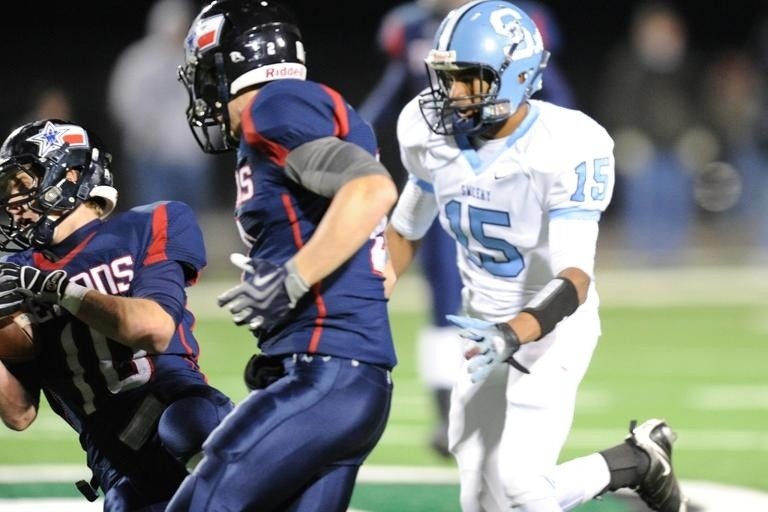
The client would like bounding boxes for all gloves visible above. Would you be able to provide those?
[216,253,310,338]
[445,314,531,384]
[0,262,70,319]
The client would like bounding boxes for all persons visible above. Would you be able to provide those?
[1,1,768,270]
[165,0,397,512]
[382,0,686,510]
[0,115,236,512]
[358,0,576,457]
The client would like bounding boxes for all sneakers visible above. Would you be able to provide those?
[625,419,687,512]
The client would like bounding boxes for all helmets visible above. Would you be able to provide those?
[184,2,308,98]
[424,1,550,124]
[0,119,118,219]
